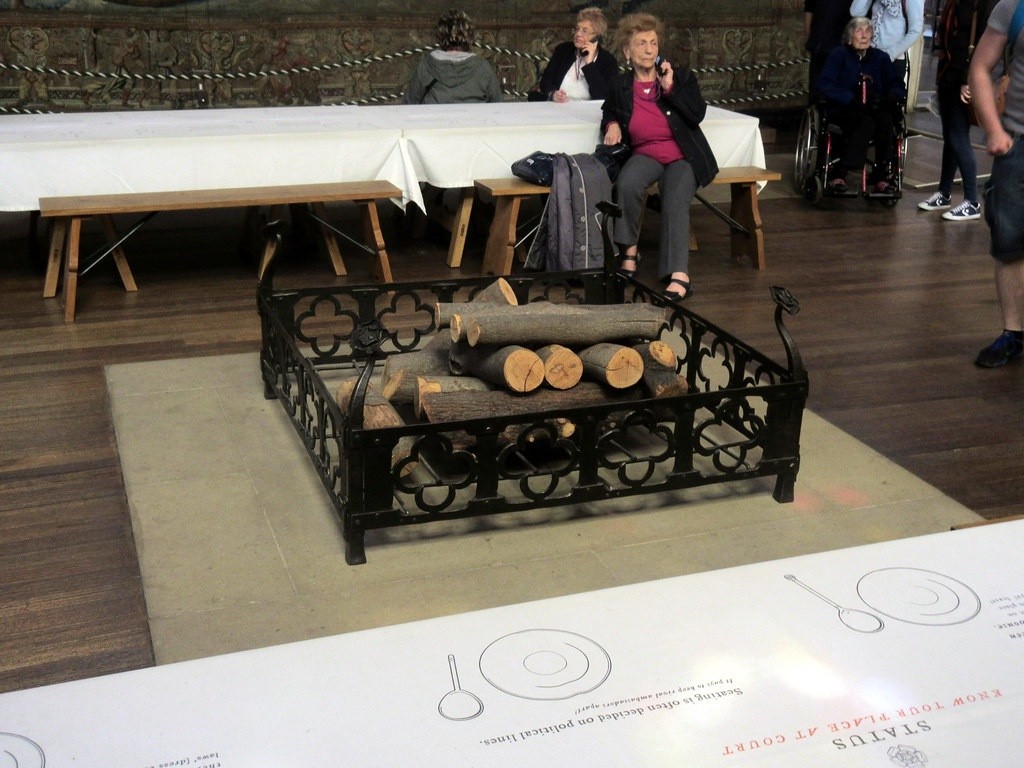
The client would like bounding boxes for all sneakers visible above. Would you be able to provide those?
[942,200,981,221]
[918,192,953,210]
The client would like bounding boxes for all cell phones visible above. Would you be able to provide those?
[655,55,668,77]
[579,34,603,57]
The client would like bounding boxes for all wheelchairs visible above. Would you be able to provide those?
[790,100,908,207]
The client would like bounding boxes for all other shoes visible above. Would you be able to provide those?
[874,181,895,194]
[972,330,1024,367]
[833,178,848,192]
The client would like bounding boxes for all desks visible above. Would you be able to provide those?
[403,100,770,268]
[0,104,428,301]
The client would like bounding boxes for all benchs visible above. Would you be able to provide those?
[37,180,403,323]
[473,164,782,279]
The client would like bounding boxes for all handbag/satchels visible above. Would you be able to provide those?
[511,151,552,186]
[940,57,970,95]
[970,75,1009,126]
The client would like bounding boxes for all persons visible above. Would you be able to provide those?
[408,9,502,104]
[918,0,991,220]
[973,0,1024,366]
[803,0,924,193]
[539,7,618,104]
[601,13,720,301]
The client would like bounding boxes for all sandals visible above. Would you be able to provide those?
[661,279,693,302]
[615,249,641,285]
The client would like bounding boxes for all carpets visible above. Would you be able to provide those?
[102,317,997,667]
